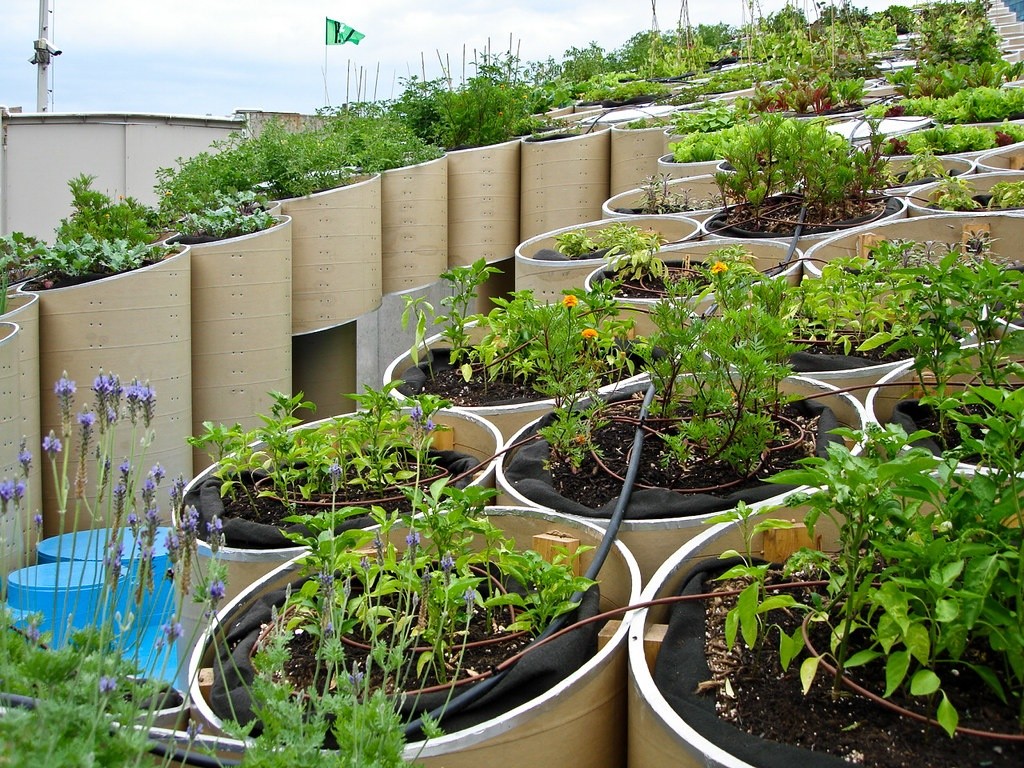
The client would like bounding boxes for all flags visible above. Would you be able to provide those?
[325,17,365,45]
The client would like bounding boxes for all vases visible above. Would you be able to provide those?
[491,366,871,591]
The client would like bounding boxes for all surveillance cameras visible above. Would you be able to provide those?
[42,39,63,56]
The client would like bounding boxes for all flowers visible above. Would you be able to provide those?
[0,366,478,768]
[511,242,847,482]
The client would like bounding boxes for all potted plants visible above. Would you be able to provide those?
[0,0,1024,768]
